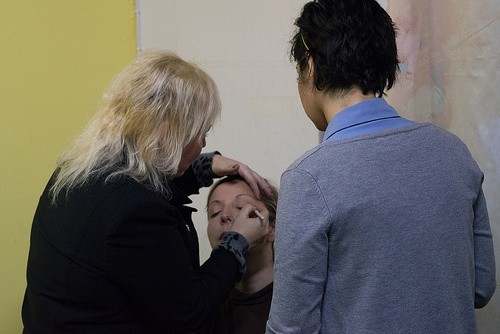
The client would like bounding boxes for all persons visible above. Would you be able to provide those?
[269,0,498,332]
[206,172,284,334]
[21,47,273,334]
